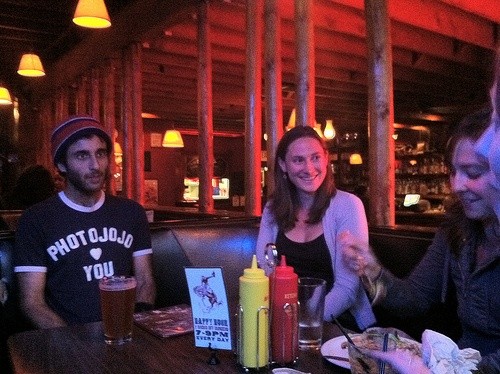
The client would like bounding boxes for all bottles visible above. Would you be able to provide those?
[395,179,452,195]
[403,158,448,173]
[268,255,299,361]
[239,254,269,368]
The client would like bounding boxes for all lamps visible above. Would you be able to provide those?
[72,0,112,29]
[0,87,13,105]
[162,124,184,148]
[17,35,46,78]
[284,107,363,166]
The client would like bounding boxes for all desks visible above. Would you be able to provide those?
[4,304,358,374]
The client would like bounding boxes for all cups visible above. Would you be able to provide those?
[99,278,137,345]
[348,334,398,374]
[298,278,327,351]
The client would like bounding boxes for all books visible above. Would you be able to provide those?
[132,302,193,341]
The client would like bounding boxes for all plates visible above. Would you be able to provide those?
[321,334,422,370]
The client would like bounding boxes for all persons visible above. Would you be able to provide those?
[366,44,500,374]
[338,101,500,346]
[256,125,377,333]
[12,113,156,330]
[0,135,58,209]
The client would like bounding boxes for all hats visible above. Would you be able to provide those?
[49,114,113,161]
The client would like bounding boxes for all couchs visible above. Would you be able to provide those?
[0,221,462,374]
